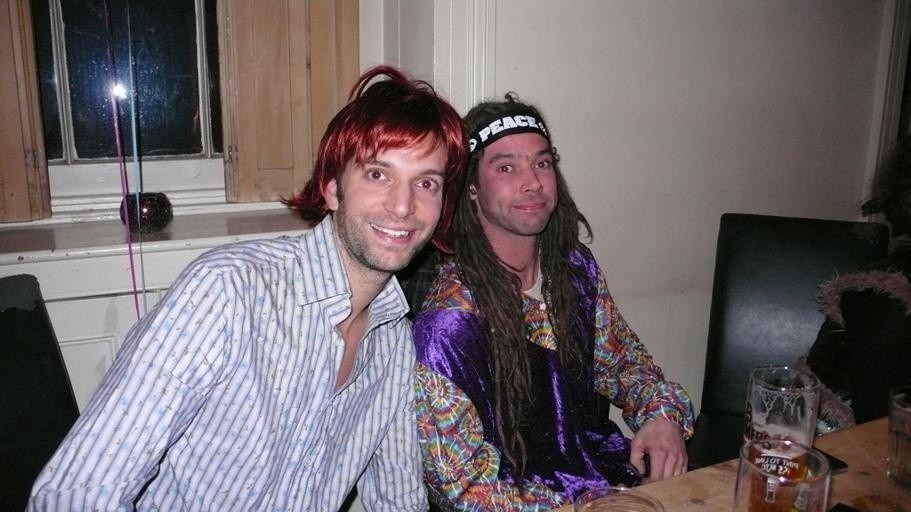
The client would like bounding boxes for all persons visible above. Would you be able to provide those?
[24,57,470,512]
[406,90,696,512]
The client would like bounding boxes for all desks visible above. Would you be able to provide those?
[538,407,911,512]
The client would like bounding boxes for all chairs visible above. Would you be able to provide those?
[1,274,83,511]
[685,212,891,471]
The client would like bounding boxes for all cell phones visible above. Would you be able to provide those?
[805,445,848,477]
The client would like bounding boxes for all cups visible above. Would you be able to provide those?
[884,383,910,490]
[732,366,832,512]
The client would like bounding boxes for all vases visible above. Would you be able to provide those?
[120,192,174,234]
